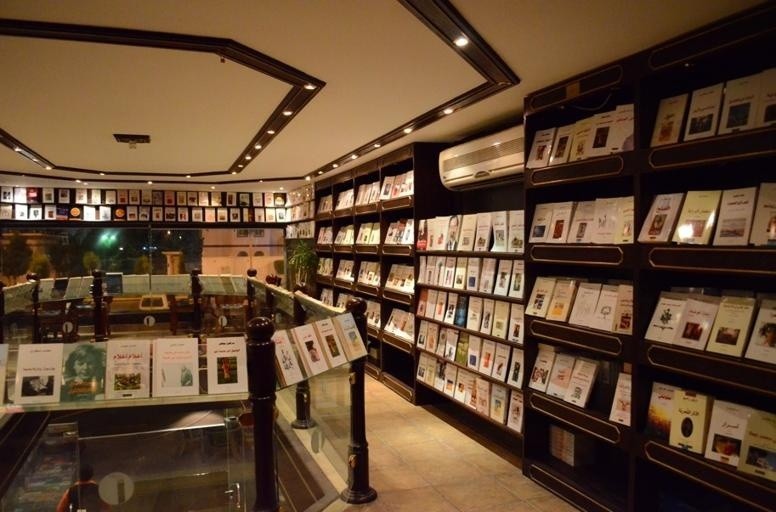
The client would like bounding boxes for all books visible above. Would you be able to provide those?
[525,275,633,336]
[643,286,776,364]
[416,211,526,299]
[528,198,634,246]
[317,257,415,345]
[636,183,774,248]
[645,379,774,481]
[526,104,634,169]
[647,72,776,149]
[152,338,248,396]
[105,272,248,296]
[414,288,525,433]
[248,278,346,319]
[270,313,369,388]
[2,277,94,314]
[15,340,151,403]
[316,170,414,244]
[548,424,575,467]
[526,343,632,428]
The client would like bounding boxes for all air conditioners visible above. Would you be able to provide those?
[439,125,523,191]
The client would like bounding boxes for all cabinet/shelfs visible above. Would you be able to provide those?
[520,53,641,511]
[412,244,524,472]
[639,3,776,511]
[318,149,475,406]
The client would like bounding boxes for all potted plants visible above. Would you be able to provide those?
[289,240,318,322]
